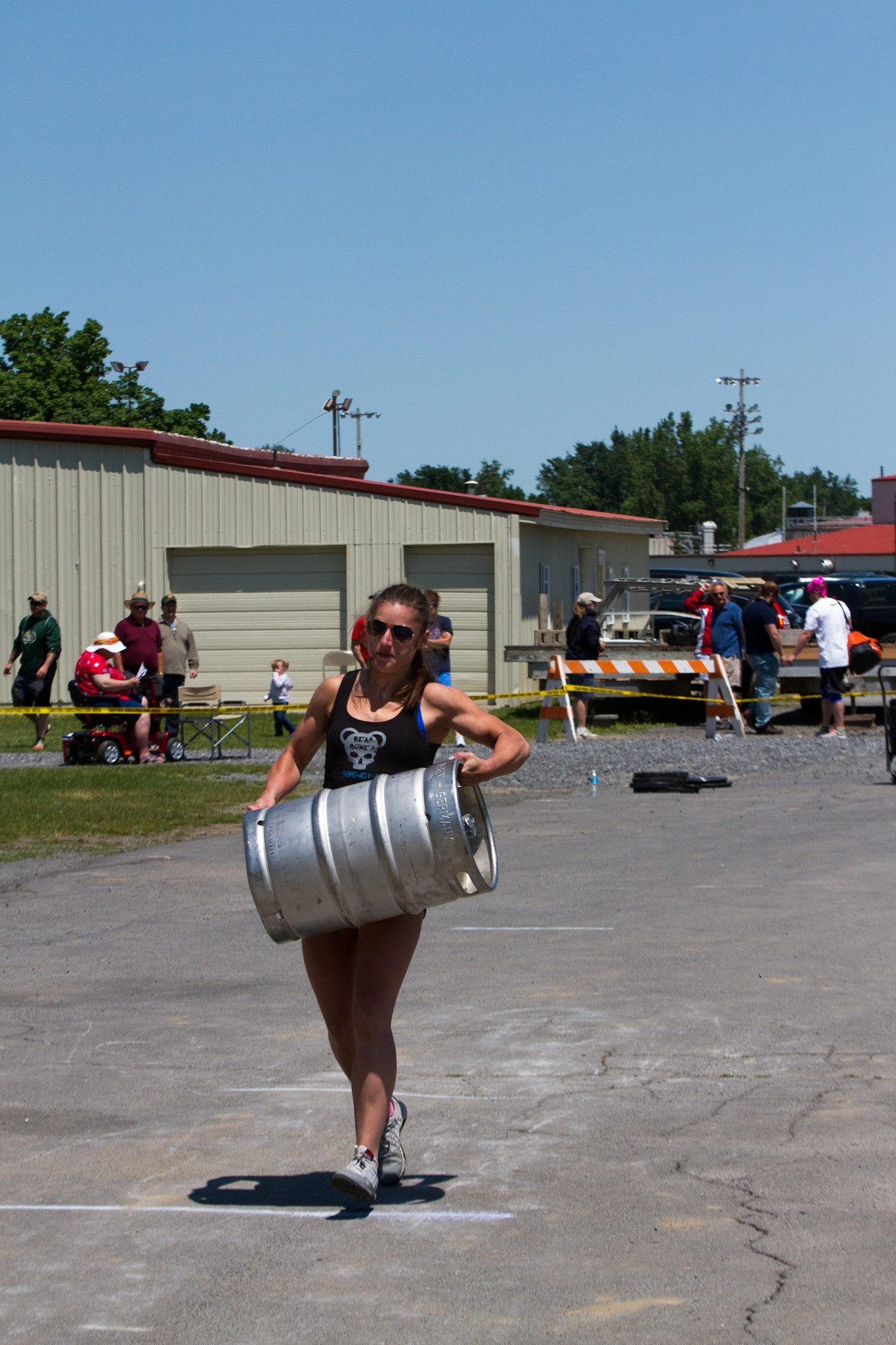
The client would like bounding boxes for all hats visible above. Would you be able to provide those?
[161,595,177,604]
[27,591,46,602]
[369,590,383,599]
[86,632,127,653]
[576,592,602,605]
[124,592,155,610]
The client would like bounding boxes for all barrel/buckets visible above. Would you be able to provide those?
[241,758,498,945]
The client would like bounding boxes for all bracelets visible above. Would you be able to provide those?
[158,672,164,675]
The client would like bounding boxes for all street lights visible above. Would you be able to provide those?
[323,389,354,456]
[715,368,764,551]
[340,408,382,459]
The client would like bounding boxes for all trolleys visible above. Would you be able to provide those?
[877,663,896,784]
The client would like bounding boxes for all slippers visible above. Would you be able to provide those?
[139,753,166,764]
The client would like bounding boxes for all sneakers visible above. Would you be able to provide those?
[332,1145,378,1212]
[819,726,847,739]
[379,1096,407,1182]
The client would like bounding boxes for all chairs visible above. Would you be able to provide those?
[179,686,254,764]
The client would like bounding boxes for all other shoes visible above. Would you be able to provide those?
[289,729,295,737]
[757,721,783,735]
[455,735,465,747]
[46,723,51,731]
[577,728,598,739]
[274,734,283,737]
[31,739,44,750]
[716,716,748,730]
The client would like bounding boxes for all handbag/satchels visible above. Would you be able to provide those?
[848,631,883,676]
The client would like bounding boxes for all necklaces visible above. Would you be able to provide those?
[371,680,394,699]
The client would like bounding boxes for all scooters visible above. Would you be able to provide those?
[61,679,184,765]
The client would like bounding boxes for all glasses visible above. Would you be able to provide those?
[429,603,438,608]
[132,605,148,610]
[30,600,45,606]
[365,619,425,644]
[712,592,724,597]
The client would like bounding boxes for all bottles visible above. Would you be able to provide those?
[589,770,598,797]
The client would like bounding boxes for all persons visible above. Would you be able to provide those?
[565,591,605,737]
[74,593,199,765]
[685,577,746,729]
[786,576,852,738]
[3,591,61,750]
[741,581,785,735]
[352,589,466,749]
[263,660,297,737]
[247,584,531,1202]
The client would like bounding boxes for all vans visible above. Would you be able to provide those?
[618,566,896,641]
